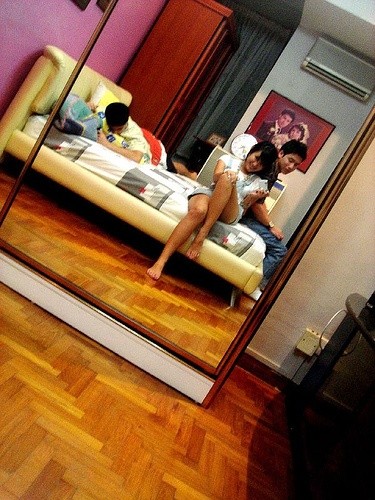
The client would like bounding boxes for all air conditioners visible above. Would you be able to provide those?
[301,36,375,102]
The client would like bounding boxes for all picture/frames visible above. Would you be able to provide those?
[206,133,226,148]
[244,90,336,174]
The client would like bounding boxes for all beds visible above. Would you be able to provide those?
[0,45,263,307]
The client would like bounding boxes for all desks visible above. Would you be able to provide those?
[284,293,375,411]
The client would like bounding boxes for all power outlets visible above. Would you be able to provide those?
[295,328,328,357]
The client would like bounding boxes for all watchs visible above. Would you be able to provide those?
[266,220,275,229]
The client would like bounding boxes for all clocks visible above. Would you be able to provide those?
[230,133,258,160]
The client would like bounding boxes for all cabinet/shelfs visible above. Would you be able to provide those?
[118,0,238,158]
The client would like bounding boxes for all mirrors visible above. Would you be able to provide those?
[1,1,375,407]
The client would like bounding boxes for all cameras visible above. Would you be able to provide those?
[259,180,269,194]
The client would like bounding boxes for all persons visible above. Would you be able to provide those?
[87,101,198,182]
[272,121,309,153]
[240,139,308,299]
[146,140,278,281]
[254,108,296,142]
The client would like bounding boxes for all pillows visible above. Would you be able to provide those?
[89,81,120,112]
